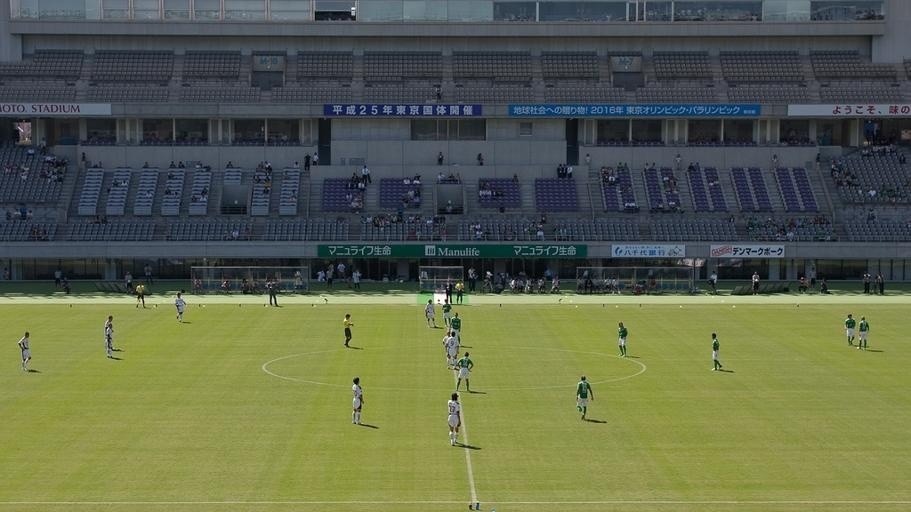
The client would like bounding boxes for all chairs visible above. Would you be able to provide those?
[1,41,911,295]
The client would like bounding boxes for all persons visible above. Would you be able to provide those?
[106,322,114,359]
[617,319,629,358]
[424,298,476,392]
[846,313,857,346]
[576,374,595,421]
[857,315,871,350]
[0,108,911,308]
[18,331,32,373]
[710,332,724,372]
[103,315,115,349]
[350,375,365,424]
[343,312,356,348]
[175,292,187,324]
[447,392,463,446]
